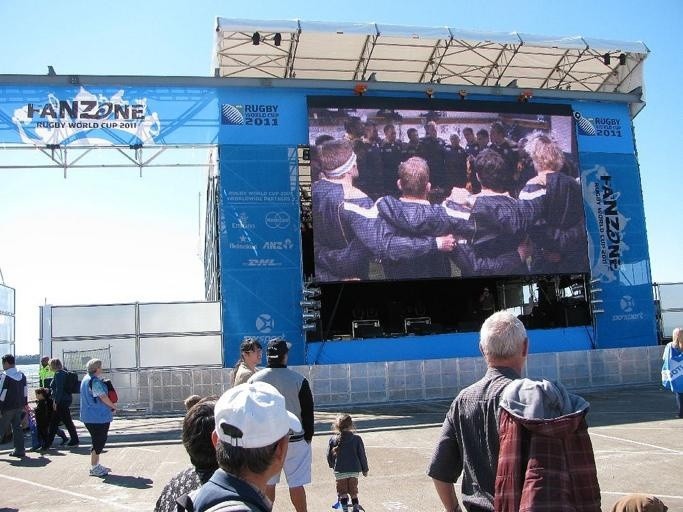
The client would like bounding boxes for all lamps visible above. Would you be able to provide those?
[604,53,611,65]
[519,90,534,103]
[589,276,606,316]
[355,82,368,96]
[620,54,627,65]
[299,281,322,333]
[252,32,260,45]
[273,33,281,46]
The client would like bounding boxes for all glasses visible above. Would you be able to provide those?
[246,349,262,353]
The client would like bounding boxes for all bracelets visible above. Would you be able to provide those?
[453,503,459,512]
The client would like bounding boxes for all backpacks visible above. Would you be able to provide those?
[89,379,118,403]
[54,370,80,393]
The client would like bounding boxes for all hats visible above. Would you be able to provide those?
[214,381,302,449]
[86,359,101,372]
[267,338,292,358]
[612,495,668,512]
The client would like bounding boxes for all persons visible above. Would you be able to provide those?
[79,358,120,476]
[154,394,221,512]
[0,352,80,458]
[660,327,683,419]
[185,395,202,411]
[232,338,262,388]
[312,115,590,285]
[326,413,369,512]
[172,381,302,512]
[247,336,314,511]
[424,310,603,512]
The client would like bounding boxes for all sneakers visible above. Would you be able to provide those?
[89,464,113,476]
[35,447,48,452]
[9,451,25,456]
[59,439,69,446]
[67,440,79,447]
[30,447,38,451]
